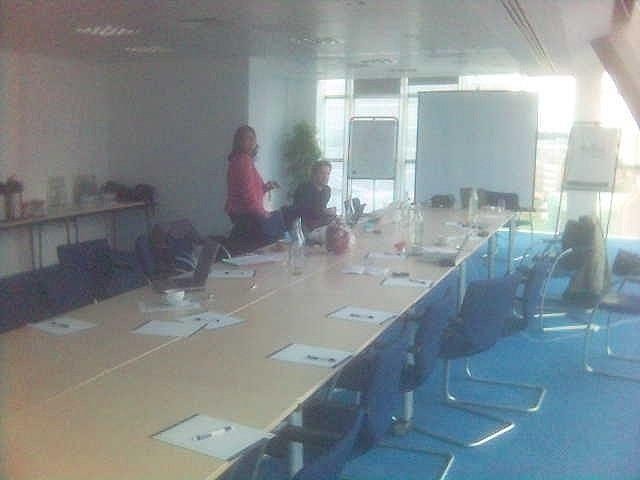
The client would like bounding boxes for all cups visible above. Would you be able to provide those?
[164,288,185,305]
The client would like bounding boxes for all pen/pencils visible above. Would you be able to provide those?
[225,272,246,275]
[350,314,375,318]
[197,426,232,440]
[307,355,336,361]
[409,279,426,284]
[48,321,70,328]
[195,318,221,322]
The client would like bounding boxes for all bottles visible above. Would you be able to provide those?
[287,215,306,277]
[468,187,480,221]
[400,198,424,256]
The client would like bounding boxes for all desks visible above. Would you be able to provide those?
[2,192,515,479]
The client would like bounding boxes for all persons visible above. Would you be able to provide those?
[223,124,281,251]
[293,159,336,231]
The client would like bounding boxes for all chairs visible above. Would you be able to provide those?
[583,251,640,381]
[442,276,547,412]
[238,404,365,479]
[399,291,520,446]
[522,245,599,337]
[319,337,451,479]
[1,192,337,331]
